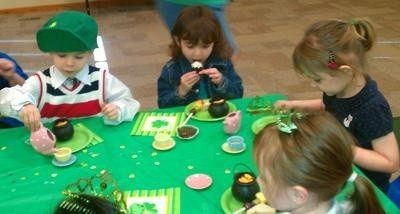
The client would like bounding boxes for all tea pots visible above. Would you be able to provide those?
[29,122,59,155]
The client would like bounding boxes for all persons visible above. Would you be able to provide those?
[154,0,236,55]
[53,191,128,214]
[157,4,244,110]
[274,17,400,196]
[251,109,387,214]
[0,9,141,133]
[0,51,29,128]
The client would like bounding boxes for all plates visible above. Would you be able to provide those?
[251,115,286,134]
[185,173,213,190]
[55,126,93,153]
[221,187,248,214]
[222,143,246,154]
[52,155,77,167]
[152,138,176,150]
[184,98,235,122]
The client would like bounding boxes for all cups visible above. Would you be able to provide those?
[227,136,244,150]
[154,134,171,148]
[56,148,71,161]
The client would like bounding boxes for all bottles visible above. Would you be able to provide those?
[233,172,259,204]
[52,118,73,142]
[208,96,229,118]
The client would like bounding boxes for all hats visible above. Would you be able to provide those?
[36,10,99,53]
